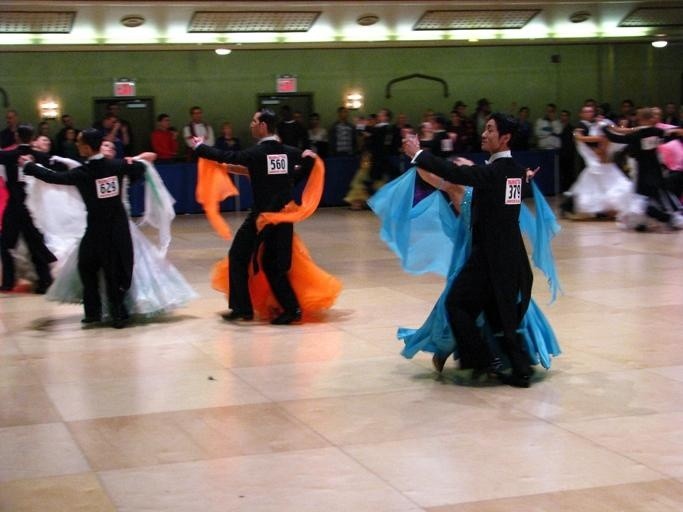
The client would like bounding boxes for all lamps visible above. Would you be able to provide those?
[215,49,231,55]
[40,103,58,119]
[651,40,668,48]
[346,94,363,110]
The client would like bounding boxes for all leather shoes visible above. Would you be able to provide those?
[221,309,253,320]
[433,350,534,388]
[271,308,301,324]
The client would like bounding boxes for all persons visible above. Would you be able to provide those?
[152,99,537,213]
[0,133,88,281]
[366,133,562,370]
[193,107,316,324]
[0,112,130,235]
[535,98,683,236]
[17,127,146,330]
[192,136,343,322]
[0,122,69,294]
[403,110,533,388]
[17,134,198,323]
